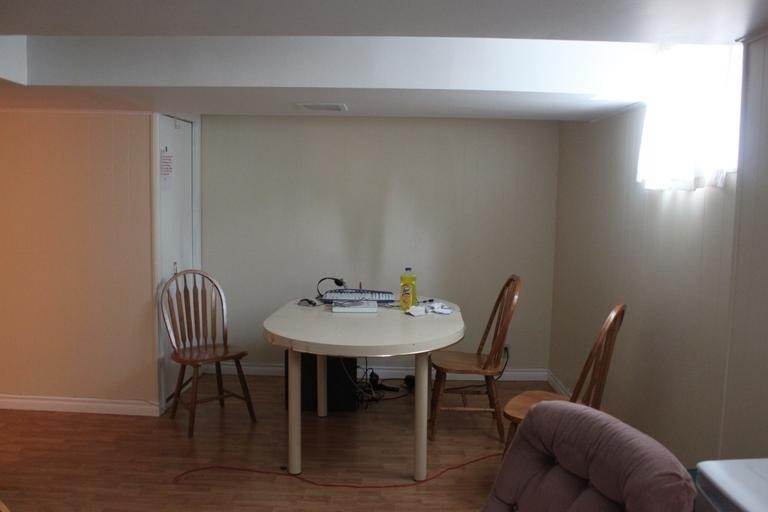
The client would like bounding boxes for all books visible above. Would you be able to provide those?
[332,299,378,313]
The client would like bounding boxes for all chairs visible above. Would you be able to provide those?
[428,272,630,461]
[476,396,699,512]
[158,268,257,441]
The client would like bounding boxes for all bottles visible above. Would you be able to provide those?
[399,266,419,312]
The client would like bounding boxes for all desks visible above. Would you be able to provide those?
[262,292,467,485]
